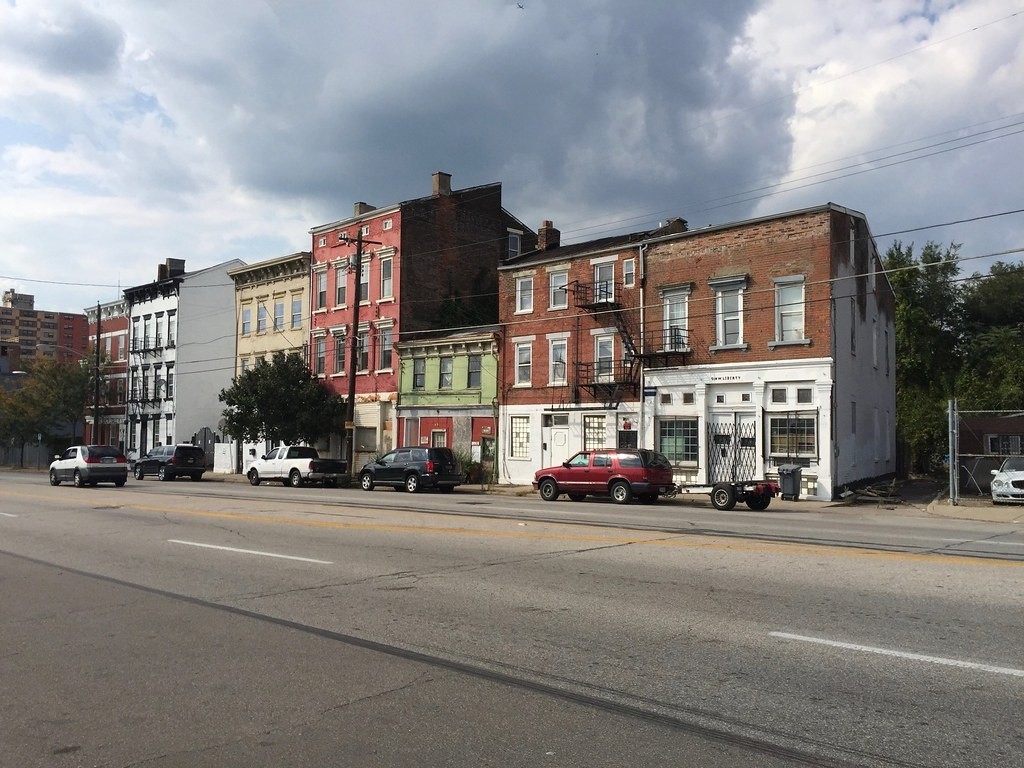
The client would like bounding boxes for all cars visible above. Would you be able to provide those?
[990,457,1024,505]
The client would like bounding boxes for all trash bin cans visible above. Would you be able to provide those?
[777,463,804,501]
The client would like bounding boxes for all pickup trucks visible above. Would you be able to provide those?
[246,444,349,489]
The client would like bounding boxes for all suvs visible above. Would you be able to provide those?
[357,445,463,493]
[133,444,208,481]
[531,448,677,505]
[49,445,128,488]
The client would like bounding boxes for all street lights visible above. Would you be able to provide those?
[36,343,101,445]
